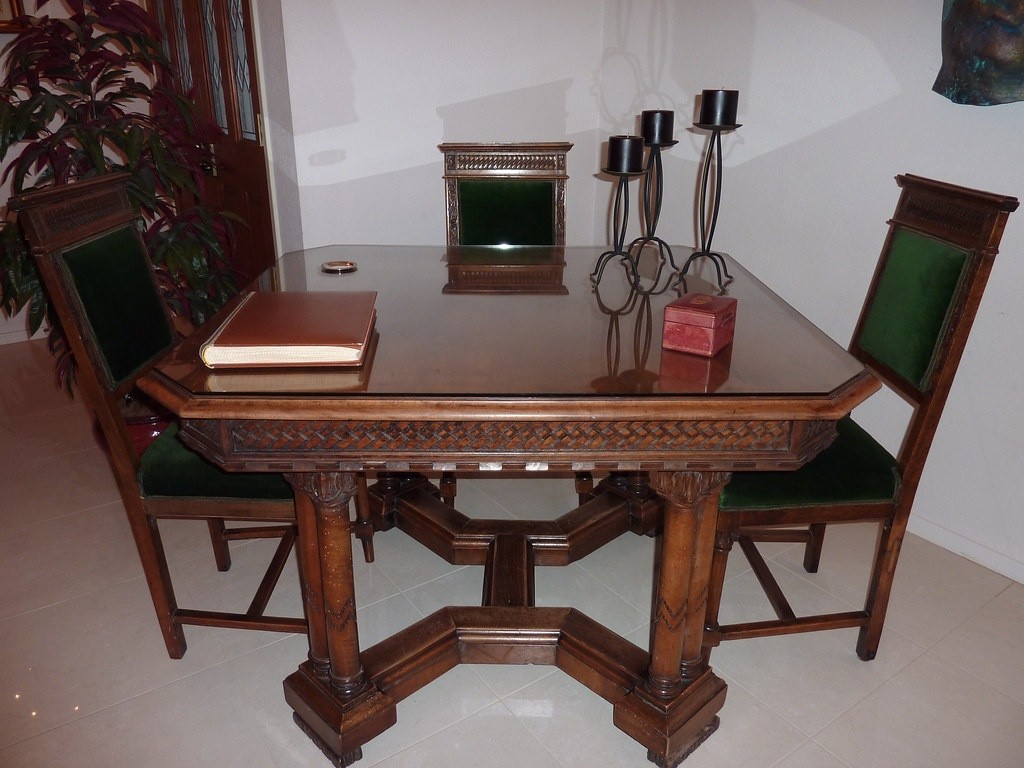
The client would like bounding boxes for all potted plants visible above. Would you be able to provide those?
[1,0,259,462]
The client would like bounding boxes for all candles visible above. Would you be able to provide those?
[608,132,643,172]
[639,109,675,144]
[700,87,740,125]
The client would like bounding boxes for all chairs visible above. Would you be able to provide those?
[439,137,595,510]
[12,171,373,661]
[701,170,1019,671]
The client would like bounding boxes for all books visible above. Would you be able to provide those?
[199,290,378,369]
[204,329,381,394]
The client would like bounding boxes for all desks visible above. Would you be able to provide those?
[137,241,884,768]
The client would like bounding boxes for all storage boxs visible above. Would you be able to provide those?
[663,289,735,358]
